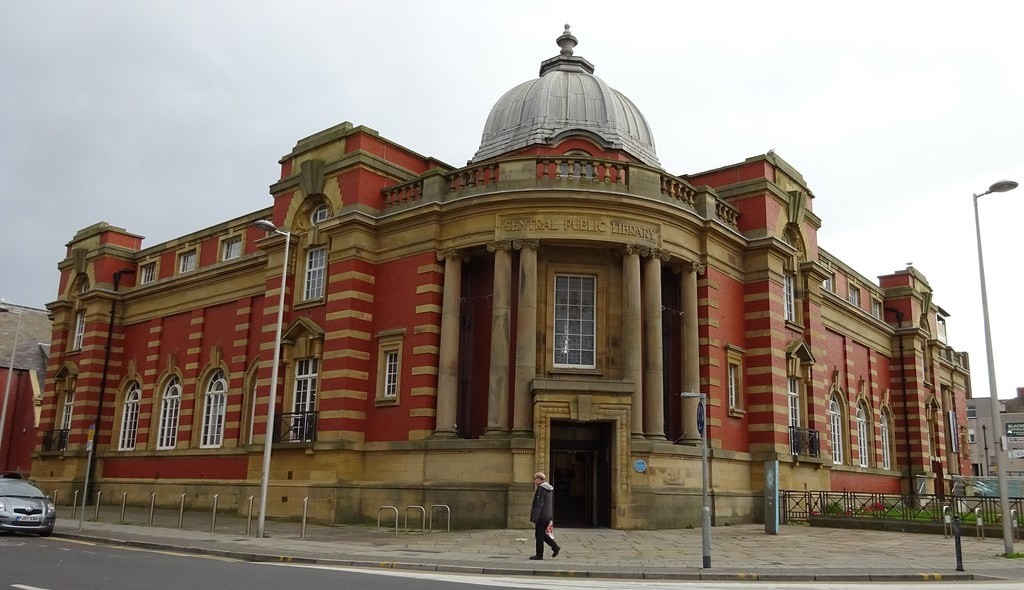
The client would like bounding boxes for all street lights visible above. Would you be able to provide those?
[973,180,1018,555]
[0,307,22,450]
[250,220,291,538]
[682,393,712,568]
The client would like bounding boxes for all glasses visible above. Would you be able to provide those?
[534,477,539,480]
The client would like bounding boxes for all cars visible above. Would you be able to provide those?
[0,478,56,537]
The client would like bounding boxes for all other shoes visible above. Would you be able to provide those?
[552,546,561,558]
[529,555,543,560]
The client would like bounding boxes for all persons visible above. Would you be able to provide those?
[529,472,561,561]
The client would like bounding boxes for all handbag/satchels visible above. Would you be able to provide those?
[545,520,555,539]
